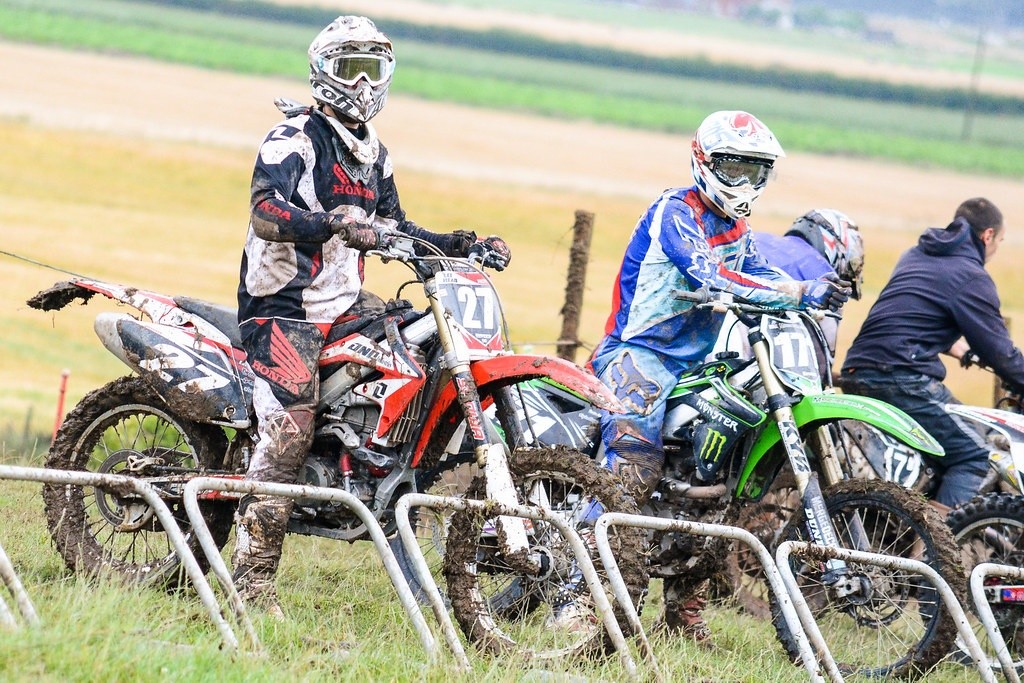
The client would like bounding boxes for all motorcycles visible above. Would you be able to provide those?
[25,215,653,674]
[440,284,1024,683]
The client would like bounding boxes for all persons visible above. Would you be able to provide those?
[229,16,512,618]
[544,109,863,656]
[841,196,1024,565]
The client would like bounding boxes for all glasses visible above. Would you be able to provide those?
[308,51,396,87]
[692,131,773,189]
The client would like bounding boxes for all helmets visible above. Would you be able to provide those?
[691,110,785,222]
[783,208,864,301]
[307,15,395,124]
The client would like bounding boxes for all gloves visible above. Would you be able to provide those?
[796,270,852,313]
[331,214,380,251]
[462,230,511,272]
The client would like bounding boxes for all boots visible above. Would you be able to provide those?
[543,518,607,639]
[908,500,956,580]
[658,598,718,655]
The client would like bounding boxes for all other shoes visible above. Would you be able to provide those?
[226,575,284,623]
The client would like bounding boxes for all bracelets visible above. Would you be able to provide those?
[960,349,975,369]
